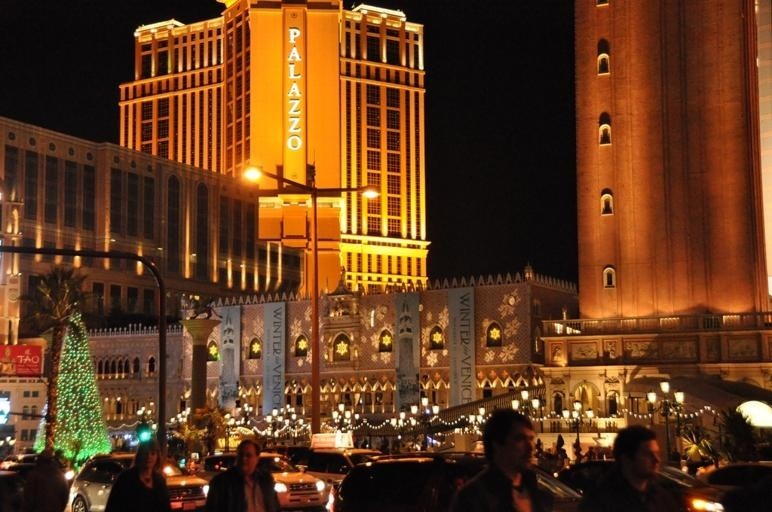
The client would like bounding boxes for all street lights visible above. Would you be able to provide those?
[246,166,381,434]
[563,398,594,451]
[136,405,153,425]
[512,388,540,418]
[386,398,440,453]
[332,399,361,433]
[0,435,17,455]
[169,406,191,431]
[220,411,234,449]
[648,380,684,462]
[470,406,485,443]
[263,403,304,448]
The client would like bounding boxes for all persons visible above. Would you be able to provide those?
[105,440,170,512]
[577,426,685,512]
[360,436,402,451]
[205,439,280,512]
[450,408,554,512]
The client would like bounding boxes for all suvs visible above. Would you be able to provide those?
[326,452,583,512]
[298,447,383,490]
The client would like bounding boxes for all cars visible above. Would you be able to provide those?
[187,452,329,509]
[554,458,744,512]
[0,453,40,472]
[694,459,772,488]
[71,453,209,512]
